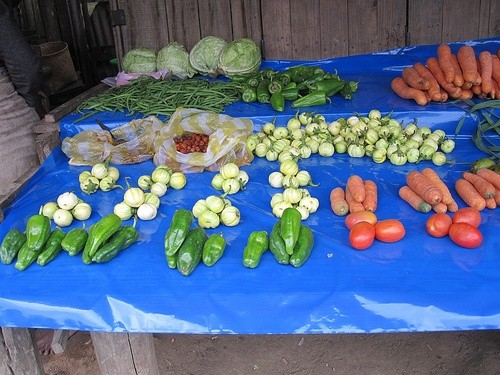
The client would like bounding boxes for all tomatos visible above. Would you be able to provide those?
[426,206,482,248]
[345,211,405,250]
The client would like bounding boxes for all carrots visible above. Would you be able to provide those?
[397,168,458,214]
[455,167,500,211]
[330,174,377,215]
[390,43,500,105]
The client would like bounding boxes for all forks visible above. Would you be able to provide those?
[96,119,125,145]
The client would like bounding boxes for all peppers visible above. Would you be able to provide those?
[242,204,315,268]
[227,65,358,112]
[0,204,139,271]
[162,208,226,276]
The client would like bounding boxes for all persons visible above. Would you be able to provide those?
[0,0,47,202]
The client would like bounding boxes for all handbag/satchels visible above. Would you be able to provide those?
[60,106,252,173]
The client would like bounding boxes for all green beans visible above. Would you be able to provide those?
[72,70,243,123]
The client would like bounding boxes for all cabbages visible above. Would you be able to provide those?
[122,36,261,79]
